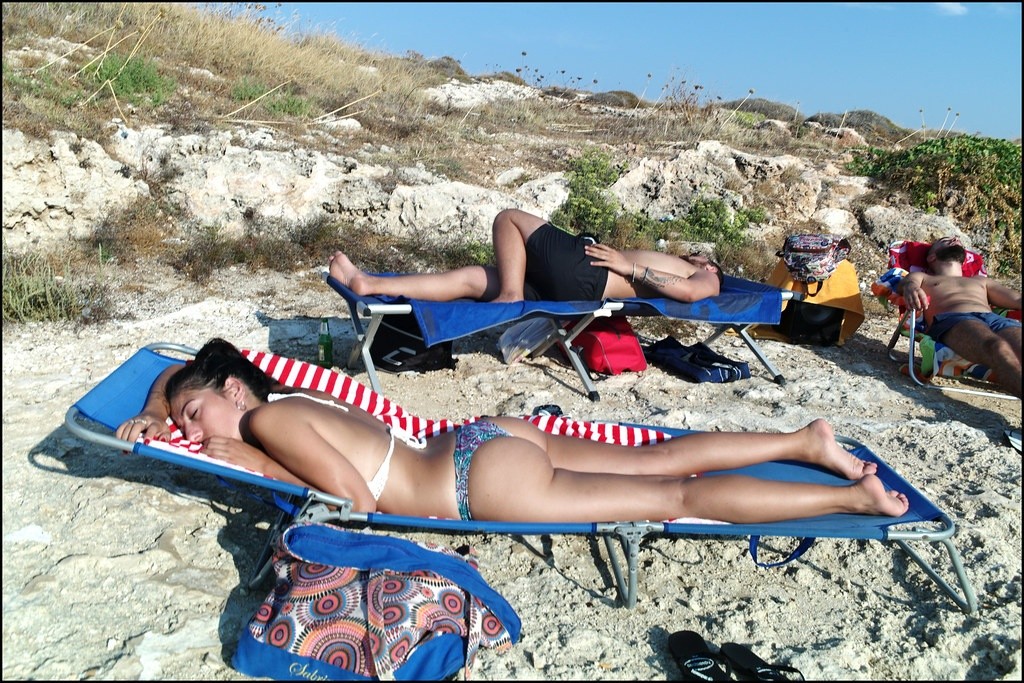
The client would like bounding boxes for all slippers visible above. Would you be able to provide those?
[668,630,806,683]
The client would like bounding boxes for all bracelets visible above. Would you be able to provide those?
[642,266,649,284]
[632,263,637,283]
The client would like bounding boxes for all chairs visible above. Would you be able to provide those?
[65,240,1022,616]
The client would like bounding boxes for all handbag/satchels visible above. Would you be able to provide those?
[561,318,646,375]
[774,235,851,298]
[230,524,522,681]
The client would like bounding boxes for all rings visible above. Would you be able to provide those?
[129,420,134,425]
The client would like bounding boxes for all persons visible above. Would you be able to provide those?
[903,236,1022,399]
[114,337,909,524]
[330,208,725,305]
